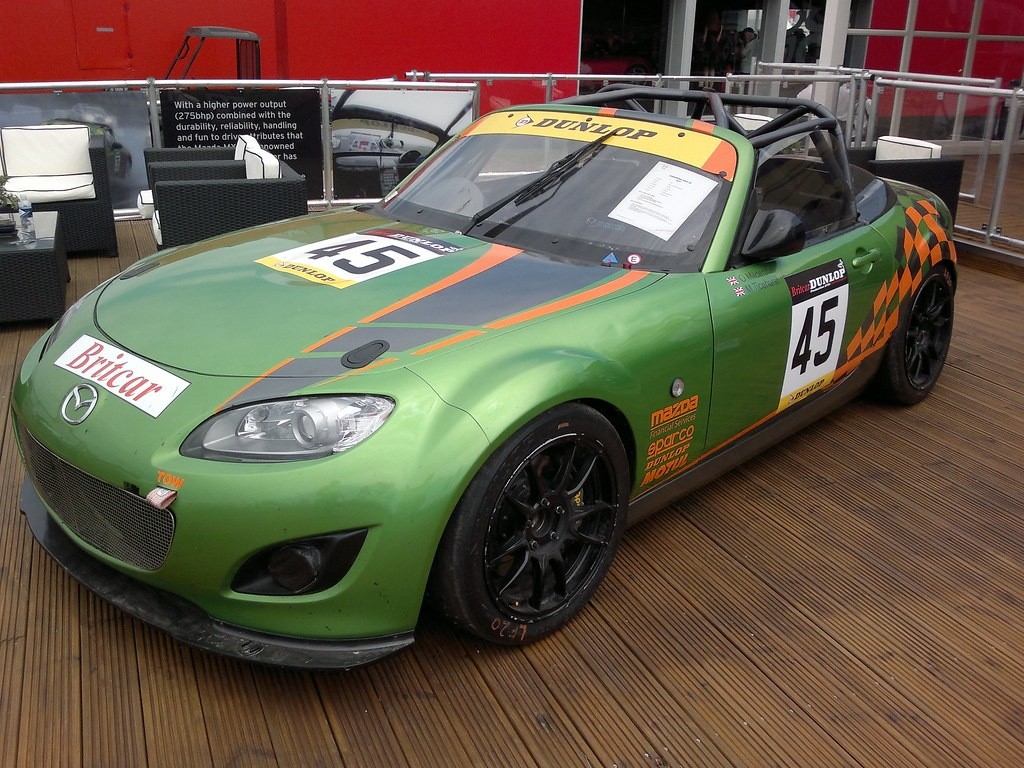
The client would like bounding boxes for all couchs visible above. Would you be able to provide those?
[136,134,308,251]
[0,124,120,259]
[808,135,966,232]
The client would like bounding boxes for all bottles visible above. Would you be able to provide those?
[18,194,36,241]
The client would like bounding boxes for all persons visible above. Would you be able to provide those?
[582,27,806,94]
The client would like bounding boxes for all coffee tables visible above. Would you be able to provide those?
[0,211,71,329]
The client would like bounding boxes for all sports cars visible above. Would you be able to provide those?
[7,83,961,670]
